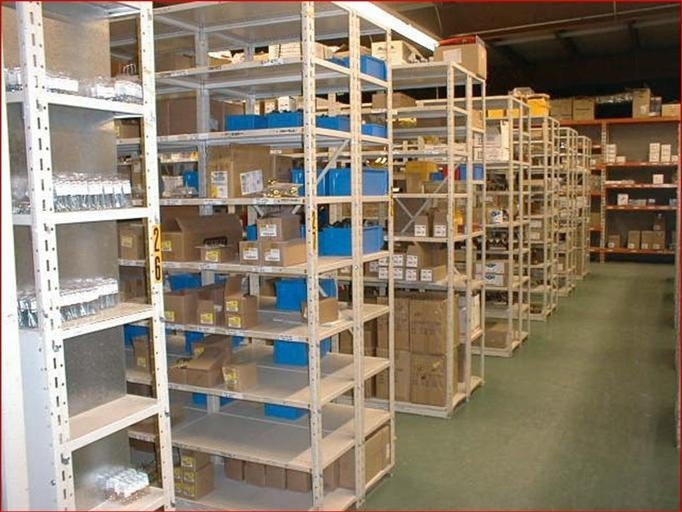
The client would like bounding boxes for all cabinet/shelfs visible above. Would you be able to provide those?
[1,0,395,512]
[376,58,592,419]
[551,88,682,265]
